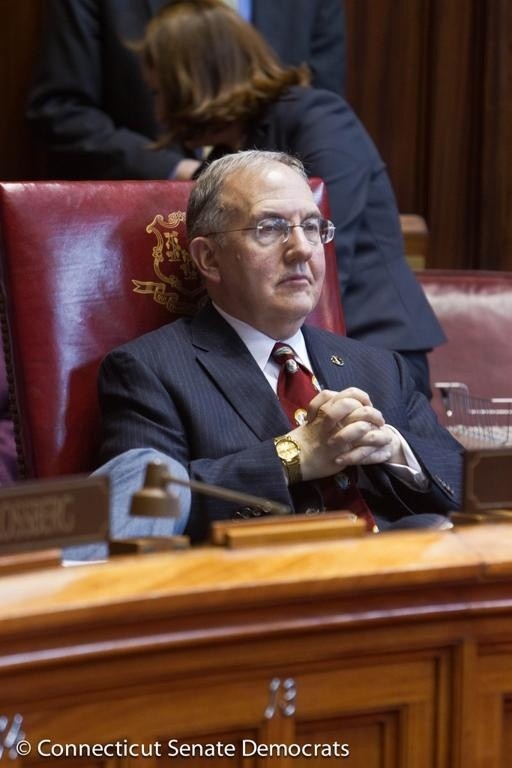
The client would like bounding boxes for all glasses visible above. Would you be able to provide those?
[202,215,337,249]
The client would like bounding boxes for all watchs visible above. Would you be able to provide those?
[273,433,305,487]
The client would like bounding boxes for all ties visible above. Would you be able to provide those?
[272,343,377,538]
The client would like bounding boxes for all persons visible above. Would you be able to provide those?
[8,1,347,179]
[91,148,474,556]
[122,0,448,406]
